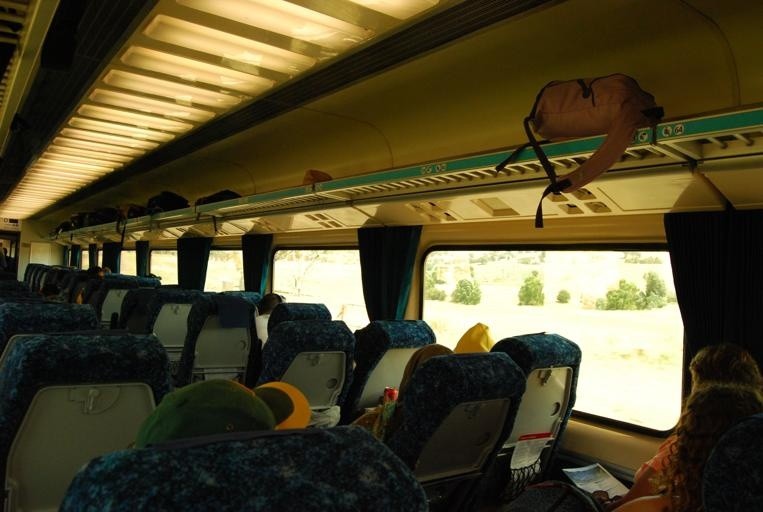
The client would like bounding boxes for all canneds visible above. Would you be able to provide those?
[384,386,399,404]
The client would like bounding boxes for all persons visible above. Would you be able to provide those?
[350,342,455,441]
[591,340,762,512]
[0,242,9,272]
[256,292,282,352]
[71,266,111,305]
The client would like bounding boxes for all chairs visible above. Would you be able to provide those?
[0,264,763,510]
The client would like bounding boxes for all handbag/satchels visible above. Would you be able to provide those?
[196,189,242,206]
[52,190,190,235]
[303,169,333,185]
[531,73,664,141]
